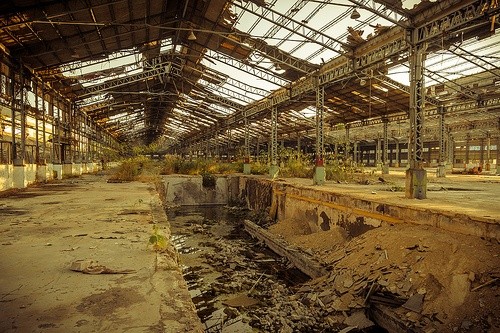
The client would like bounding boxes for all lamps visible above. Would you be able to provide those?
[350,7,361,19]
[187,30,197,40]
[360,79,366,86]
